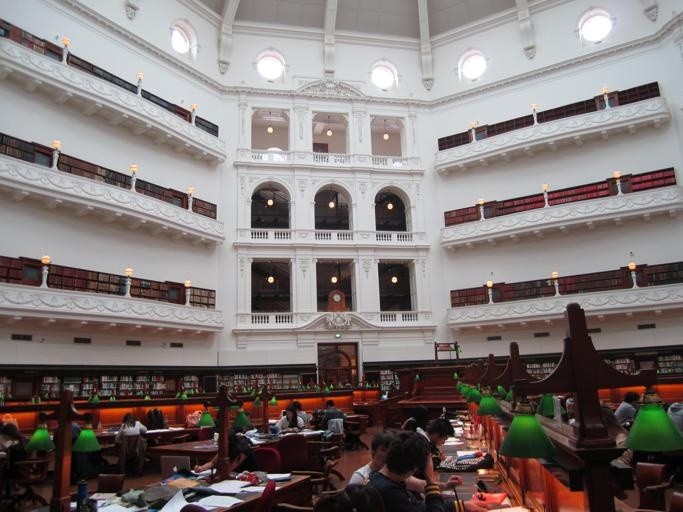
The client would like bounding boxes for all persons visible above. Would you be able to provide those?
[72,423,82,440]
[320,400,343,428]
[613,391,640,432]
[194,427,256,484]
[0,422,32,503]
[519,395,535,412]
[347,404,491,511]
[273,405,305,434]
[292,401,310,424]
[115,413,148,478]
[600,405,633,466]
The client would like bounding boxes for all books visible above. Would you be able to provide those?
[265,472,292,482]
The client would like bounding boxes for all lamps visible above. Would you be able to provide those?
[454,372,683,458]
[267,112,398,283]
[315,383,384,395]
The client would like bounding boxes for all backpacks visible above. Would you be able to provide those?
[146,407,165,430]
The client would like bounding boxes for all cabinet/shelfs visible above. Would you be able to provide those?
[438,81,661,152]
[444,166,676,228]
[0,132,217,219]
[0,255,216,309]
[0,17,218,138]
[450,261,683,308]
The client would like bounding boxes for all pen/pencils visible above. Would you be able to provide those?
[241,484,253,488]
[452,486,458,501]
[196,457,199,468]
